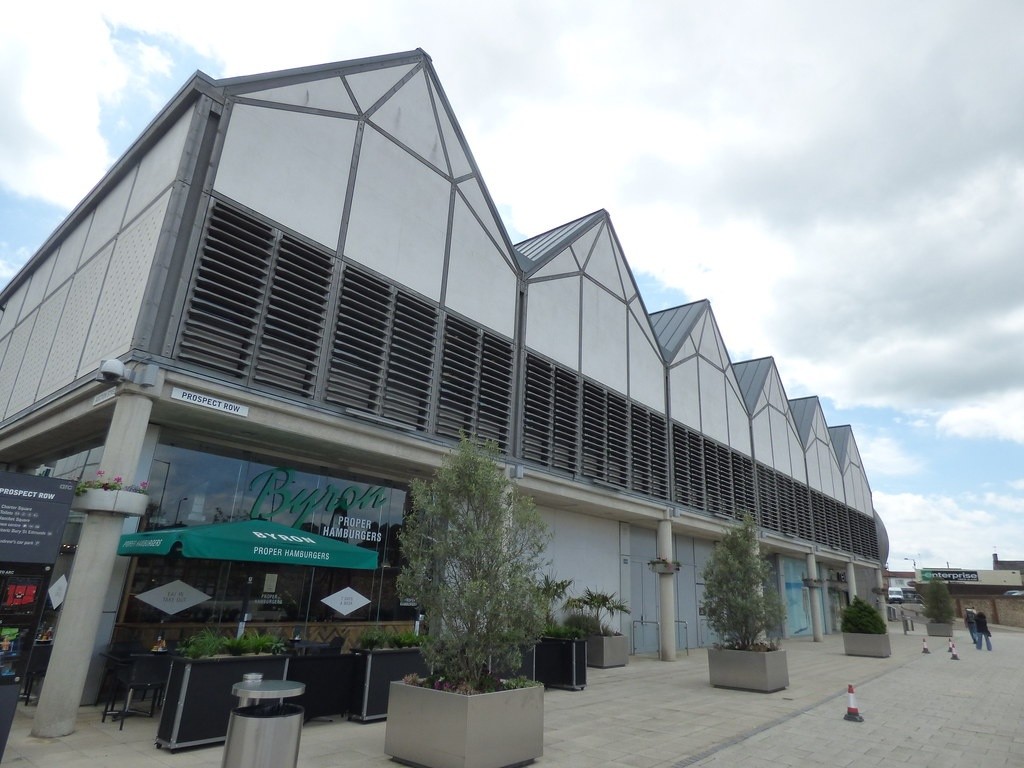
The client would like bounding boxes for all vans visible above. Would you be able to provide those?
[888,587,904,604]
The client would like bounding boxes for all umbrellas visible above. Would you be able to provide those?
[117,519,378,639]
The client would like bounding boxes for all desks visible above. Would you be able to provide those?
[285,637,329,655]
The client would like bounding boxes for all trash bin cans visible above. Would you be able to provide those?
[222,680,306,768]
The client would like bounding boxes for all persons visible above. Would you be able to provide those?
[964,609,977,643]
[974,612,992,651]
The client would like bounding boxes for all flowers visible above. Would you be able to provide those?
[74,469,149,495]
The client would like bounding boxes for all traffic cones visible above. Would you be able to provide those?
[947,638,955,652]
[843,684,864,722]
[951,644,960,660]
[921,638,930,653]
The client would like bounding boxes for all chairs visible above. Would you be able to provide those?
[96,636,345,731]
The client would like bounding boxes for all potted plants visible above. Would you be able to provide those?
[647,557,681,574]
[347,427,631,768]
[915,576,958,637]
[698,511,790,694]
[179,627,286,659]
[840,595,891,658]
